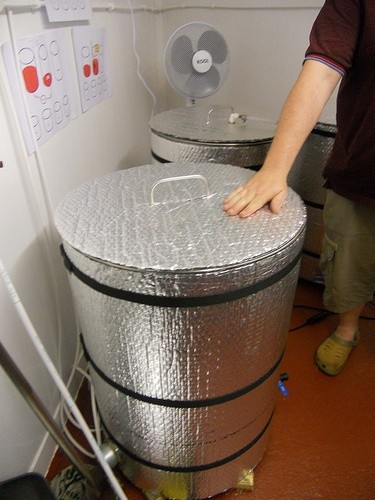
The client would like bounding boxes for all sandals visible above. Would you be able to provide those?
[316,326,361,376]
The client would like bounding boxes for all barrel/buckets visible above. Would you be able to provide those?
[305,122,336,258]
[54,162,307,500]
[148,105,277,193]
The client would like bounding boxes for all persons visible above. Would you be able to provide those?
[223,0,375,376]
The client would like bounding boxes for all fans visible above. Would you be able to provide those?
[163,21,230,107]
